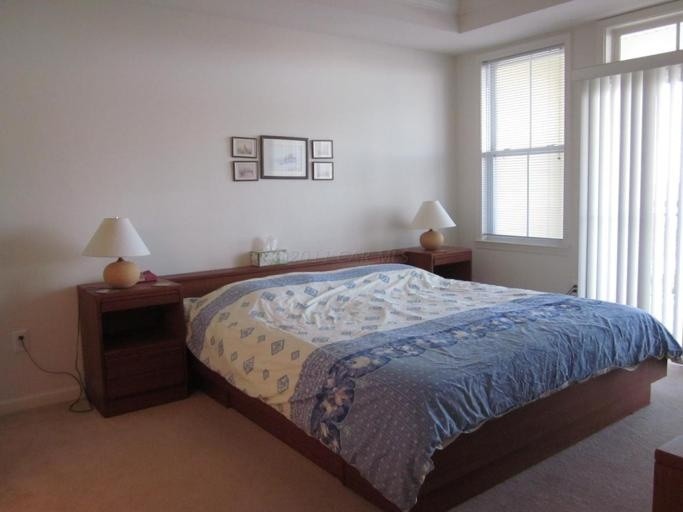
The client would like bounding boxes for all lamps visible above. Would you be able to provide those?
[408,200,456,250]
[81,215,152,288]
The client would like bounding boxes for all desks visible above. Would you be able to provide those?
[652,434,683,512]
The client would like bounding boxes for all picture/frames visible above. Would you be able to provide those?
[232,135,334,181]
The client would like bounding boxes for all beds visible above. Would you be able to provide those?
[158,248,668,512]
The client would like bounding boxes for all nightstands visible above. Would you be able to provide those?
[404,246,472,281]
[77,277,190,418]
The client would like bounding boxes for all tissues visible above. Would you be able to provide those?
[248,237,288,268]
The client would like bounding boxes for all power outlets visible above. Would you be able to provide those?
[10,328,29,353]
